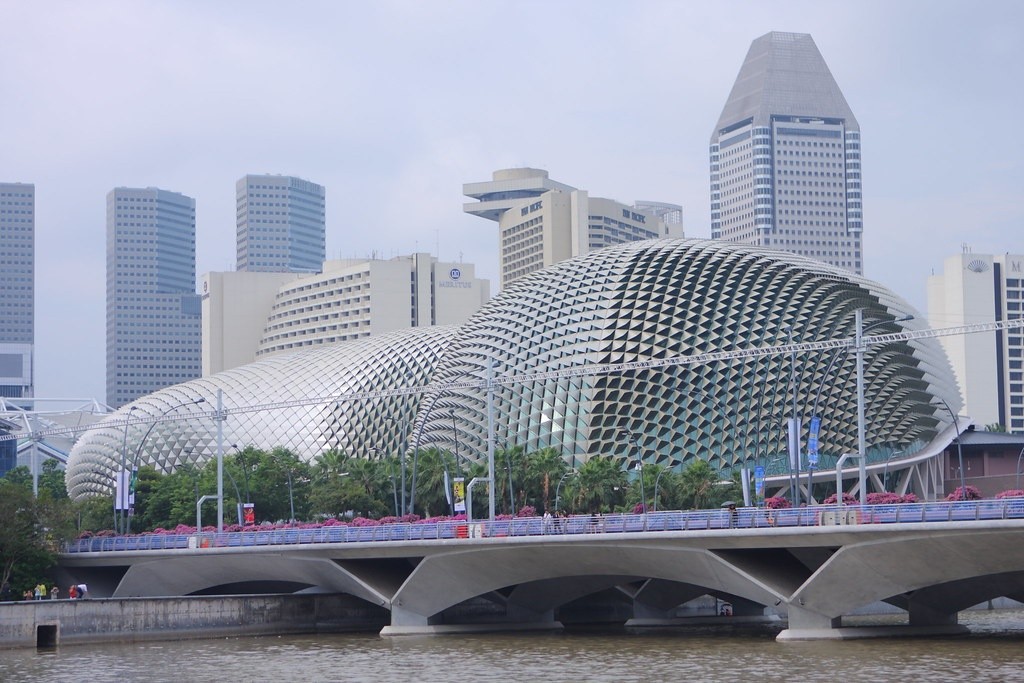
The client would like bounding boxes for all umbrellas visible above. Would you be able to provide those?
[721,501,736,506]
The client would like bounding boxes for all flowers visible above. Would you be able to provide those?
[495,504,536,520]
[632,502,654,514]
[80,513,467,538]
[763,486,1024,509]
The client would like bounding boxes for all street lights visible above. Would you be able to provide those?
[410,361,502,514]
[372,447,399,518]
[613,426,646,515]
[653,465,675,511]
[126,397,206,535]
[384,415,454,519]
[230,444,250,504]
[401,373,414,516]
[806,315,916,509]
[448,409,466,514]
[270,455,296,527]
[785,326,802,509]
[184,450,244,527]
[120,406,138,537]
[671,386,752,507]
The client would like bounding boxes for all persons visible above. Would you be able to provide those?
[25,590,32,600]
[590,511,604,534]
[765,503,774,528]
[69,583,87,599]
[58,540,66,554]
[52,588,59,599]
[35,583,46,600]
[729,505,738,530]
[544,510,563,535]
